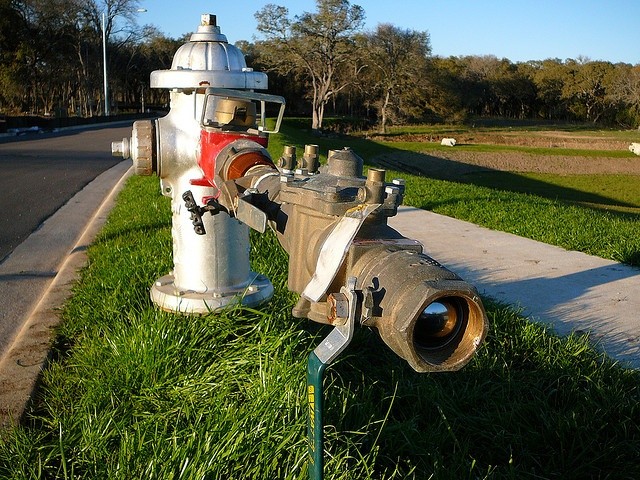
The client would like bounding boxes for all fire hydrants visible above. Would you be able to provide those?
[111,14,273,318]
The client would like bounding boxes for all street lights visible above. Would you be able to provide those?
[102,9,148,116]
